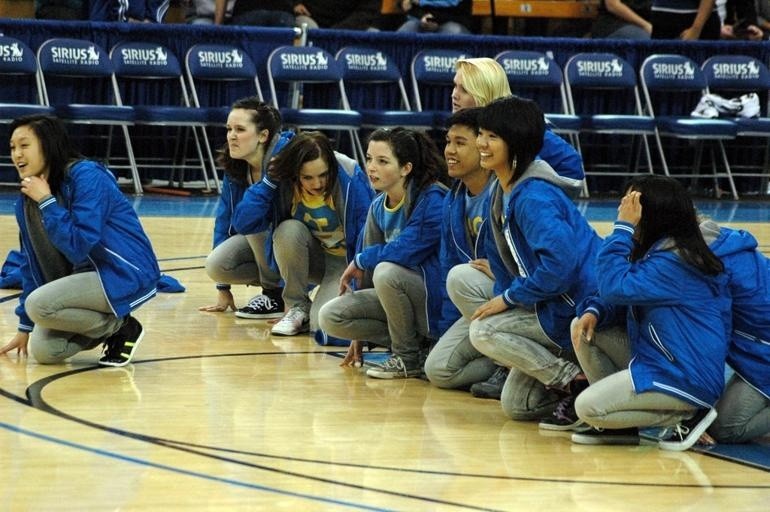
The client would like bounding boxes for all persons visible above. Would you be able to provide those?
[83,1,476,182]
[0,114,162,369]
[205,93,769,454]
[586,1,769,41]
[452,57,586,180]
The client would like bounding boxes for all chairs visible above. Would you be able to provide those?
[0,34,46,188]
[335,47,436,168]
[495,50,590,199]
[179,43,264,196]
[37,38,141,195]
[411,48,477,132]
[633,53,740,202]
[106,41,212,197]
[266,45,375,192]
[693,55,770,201]
[561,52,654,200]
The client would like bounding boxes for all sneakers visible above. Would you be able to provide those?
[571,425,640,445]
[418,338,437,379]
[539,386,585,431]
[658,407,718,451]
[470,366,510,398]
[367,354,421,378]
[99,317,144,367]
[272,307,309,336]
[235,295,285,319]
